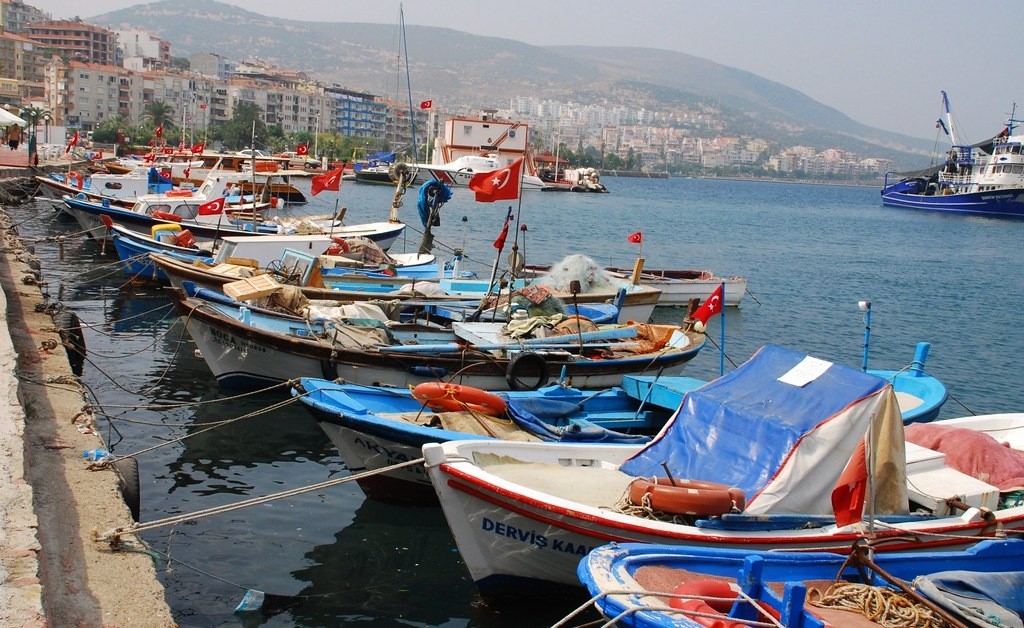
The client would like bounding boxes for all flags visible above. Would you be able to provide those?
[397,112,403,117]
[310,166,344,196]
[628,231,642,244]
[65,104,310,216]
[420,100,432,110]
[689,286,722,327]
[493,223,509,253]
[831,422,872,527]
[468,156,525,203]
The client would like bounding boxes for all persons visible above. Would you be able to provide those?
[6,123,20,152]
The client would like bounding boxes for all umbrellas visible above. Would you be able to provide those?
[1,108,27,128]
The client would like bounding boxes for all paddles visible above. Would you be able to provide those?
[377,323,639,351]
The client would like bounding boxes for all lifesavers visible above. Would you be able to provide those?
[993,156,999,162]
[671,577,836,628]
[65,171,83,190]
[629,475,746,513]
[1001,135,1007,143]
[324,236,350,256]
[413,381,506,417]
[152,210,183,222]
[165,189,193,197]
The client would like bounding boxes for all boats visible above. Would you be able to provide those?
[163,206,707,411]
[298,280,951,517]
[575,414,1022,628]
[419,341,1021,623]
[32,1,748,309]
[881,87,1023,219]
